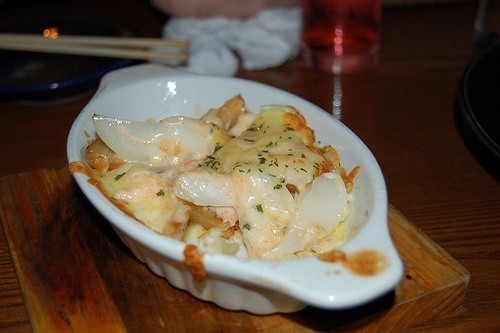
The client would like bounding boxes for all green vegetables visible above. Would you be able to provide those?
[113,120,320,229]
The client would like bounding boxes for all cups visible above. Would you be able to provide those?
[298,0,383,74]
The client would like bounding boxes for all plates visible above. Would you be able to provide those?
[454,39,500,171]
[0,50,131,94]
[67,62,405,315]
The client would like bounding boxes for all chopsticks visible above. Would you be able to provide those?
[0,32,189,62]
